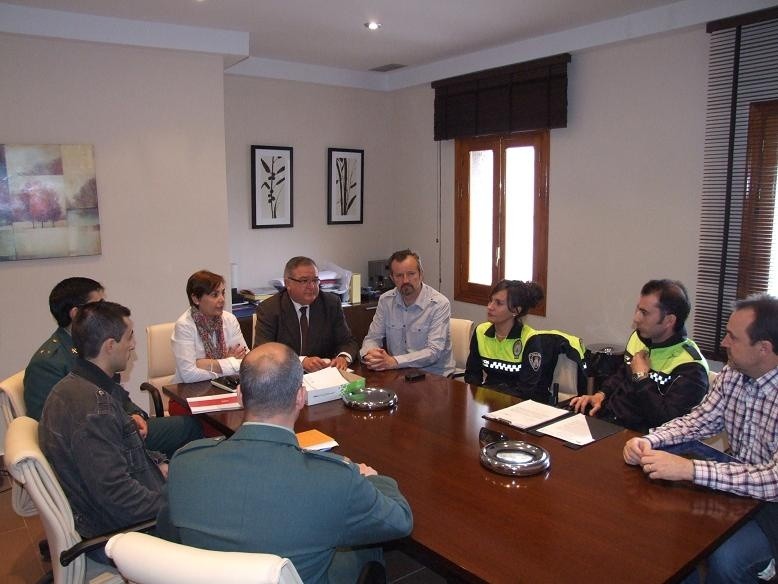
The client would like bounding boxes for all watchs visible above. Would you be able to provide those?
[132,411,147,421]
[338,354,351,362]
[632,372,648,382]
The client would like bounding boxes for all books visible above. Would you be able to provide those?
[186,393,243,414]
[211,375,240,392]
[232,288,279,318]
[296,428,339,449]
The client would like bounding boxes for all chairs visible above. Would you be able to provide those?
[106,530,387,584]
[141,322,176,417]
[2,415,160,584]
[0,369,39,517]
[447,318,475,382]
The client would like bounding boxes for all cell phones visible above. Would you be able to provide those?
[405,374,425,381]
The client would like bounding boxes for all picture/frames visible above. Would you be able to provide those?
[250,145,293,228]
[327,147,363,225]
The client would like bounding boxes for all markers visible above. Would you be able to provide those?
[365,307,377,311]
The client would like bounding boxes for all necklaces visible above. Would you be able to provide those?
[496,333,506,337]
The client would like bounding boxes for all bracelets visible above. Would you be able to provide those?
[210,359,212,371]
[197,360,199,367]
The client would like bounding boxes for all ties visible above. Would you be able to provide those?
[299,305,309,355]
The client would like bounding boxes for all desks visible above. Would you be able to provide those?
[237,300,379,350]
[163,359,764,584]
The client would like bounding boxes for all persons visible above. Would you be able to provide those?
[464,280,544,400]
[623,293,778,584]
[23,277,204,462]
[38,301,169,567]
[168,270,250,440]
[569,279,710,435]
[358,249,456,378]
[153,342,413,584]
[252,257,359,372]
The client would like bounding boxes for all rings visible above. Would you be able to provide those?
[648,464,652,472]
[375,364,376,369]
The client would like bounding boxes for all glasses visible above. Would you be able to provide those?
[288,277,321,286]
